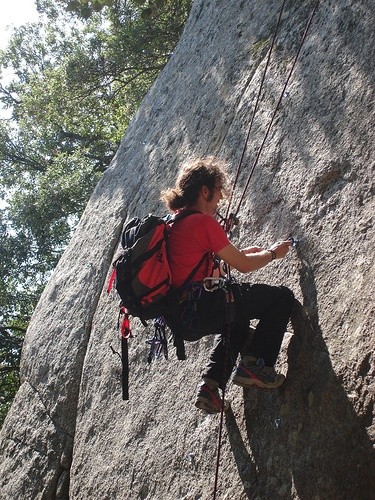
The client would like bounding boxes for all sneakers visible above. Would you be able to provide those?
[194,384,229,414]
[233,362,285,389]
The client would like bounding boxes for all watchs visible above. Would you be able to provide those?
[267,249,277,261]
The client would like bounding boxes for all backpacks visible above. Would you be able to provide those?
[112,210,210,320]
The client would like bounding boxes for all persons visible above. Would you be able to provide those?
[159,161,295,414]
